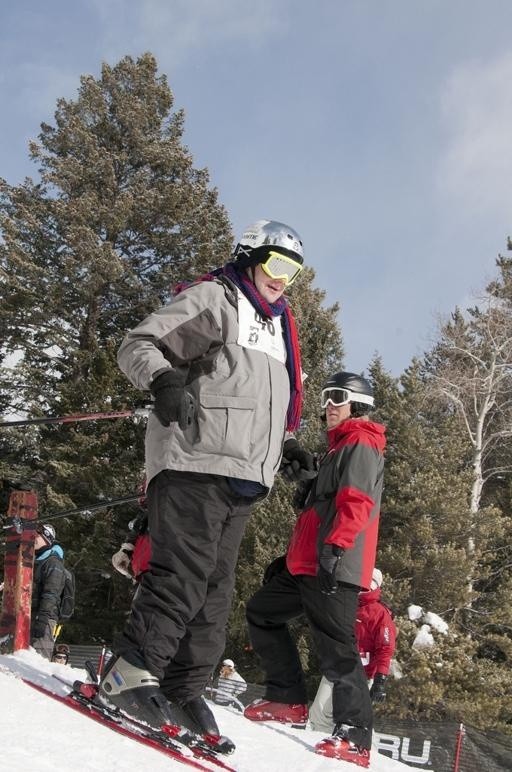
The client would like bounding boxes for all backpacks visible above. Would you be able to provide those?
[36,555,76,619]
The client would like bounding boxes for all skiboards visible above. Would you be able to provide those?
[1,657,238,772]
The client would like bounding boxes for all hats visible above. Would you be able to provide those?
[224,659,235,670]
[52,643,69,665]
[369,567,383,591]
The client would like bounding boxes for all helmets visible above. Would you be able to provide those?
[322,372,375,415]
[37,523,57,547]
[234,220,304,264]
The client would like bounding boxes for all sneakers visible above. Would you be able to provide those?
[314,735,370,769]
[98,653,181,739]
[243,699,309,724]
[167,695,221,749]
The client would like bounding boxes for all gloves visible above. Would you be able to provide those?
[32,621,45,637]
[112,542,134,578]
[317,545,344,597]
[281,439,321,482]
[150,371,196,430]
[369,673,388,704]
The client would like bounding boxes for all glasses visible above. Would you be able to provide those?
[239,250,303,286]
[55,655,67,660]
[320,387,376,409]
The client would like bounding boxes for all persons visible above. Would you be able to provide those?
[240,371,388,769]
[95,216,302,756]
[28,522,68,663]
[50,643,71,666]
[306,568,395,734]
[211,658,248,708]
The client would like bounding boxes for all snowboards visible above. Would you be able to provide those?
[0,490,38,655]
